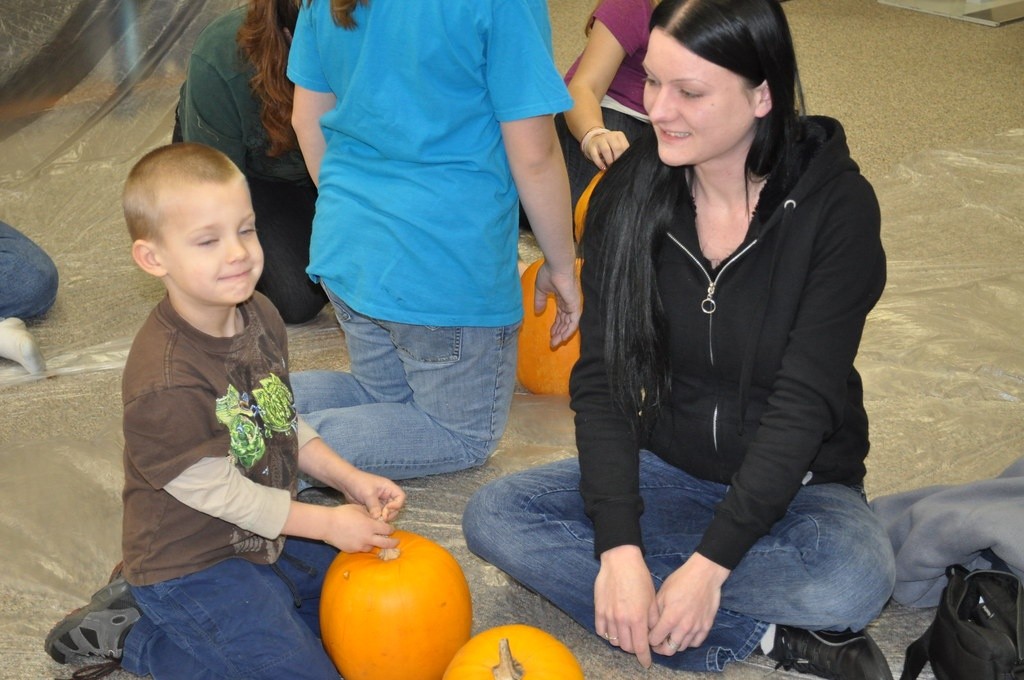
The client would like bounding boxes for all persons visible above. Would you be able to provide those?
[462,1,892,679]
[287,0,583,493]
[519,0,652,235]
[44,143,407,679]
[172,1,328,324]
[0,222,59,388]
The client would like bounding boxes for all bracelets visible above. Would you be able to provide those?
[581,124,610,161]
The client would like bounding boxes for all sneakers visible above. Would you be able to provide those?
[45,560,143,680]
[765,620,894,680]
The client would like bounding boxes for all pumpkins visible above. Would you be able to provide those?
[573,169,603,243]
[441,621,584,680]
[518,253,584,396]
[321,525,473,680]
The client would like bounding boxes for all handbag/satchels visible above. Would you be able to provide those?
[899,548,1024,680]
